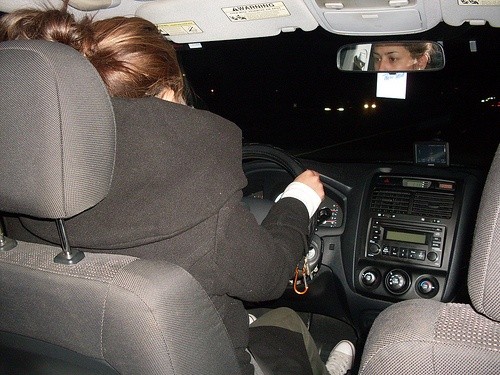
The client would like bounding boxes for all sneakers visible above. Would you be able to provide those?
[248,313,256,325]
[326,340,355,375]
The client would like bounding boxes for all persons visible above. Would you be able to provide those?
[372,42,435,71]
[0,5,355,375]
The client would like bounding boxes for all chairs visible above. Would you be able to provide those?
[0,39,242,375]
[360,145,500,375]
